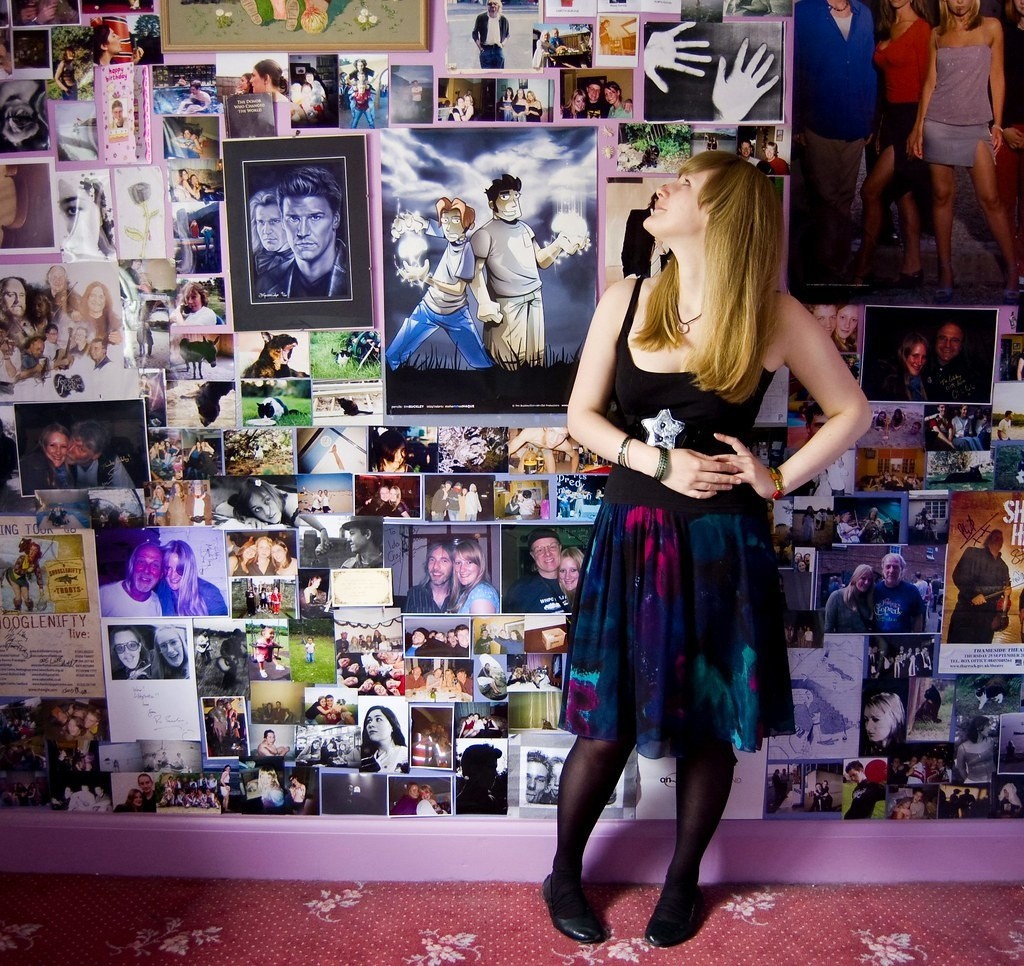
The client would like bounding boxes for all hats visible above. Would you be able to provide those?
[341,516,384,530]
[527,527,560,546]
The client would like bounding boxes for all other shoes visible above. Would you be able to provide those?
[275,664,285,671]
[844,268,879,297]
[898,267,924,289]
[260,669,269,678]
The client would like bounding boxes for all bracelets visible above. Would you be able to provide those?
[618,435,633,469]
[653,445,668,483]
[768,465,784,500]
[993,124,1004,132]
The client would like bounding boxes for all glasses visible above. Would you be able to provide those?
[154,637,183,655]
[531,542,560,556]
[114,641,141,655]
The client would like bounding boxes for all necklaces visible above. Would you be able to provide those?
[676,304,701,333]
[827,0,848,12]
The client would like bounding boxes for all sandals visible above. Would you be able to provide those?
[934,286,953,301]
[1003,287,1020,304]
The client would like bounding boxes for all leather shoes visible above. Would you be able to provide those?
[644,887,705,947]
[542,873,606,944]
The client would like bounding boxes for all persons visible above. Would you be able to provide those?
[0,0,1024,823]
[542,151,875,948]
